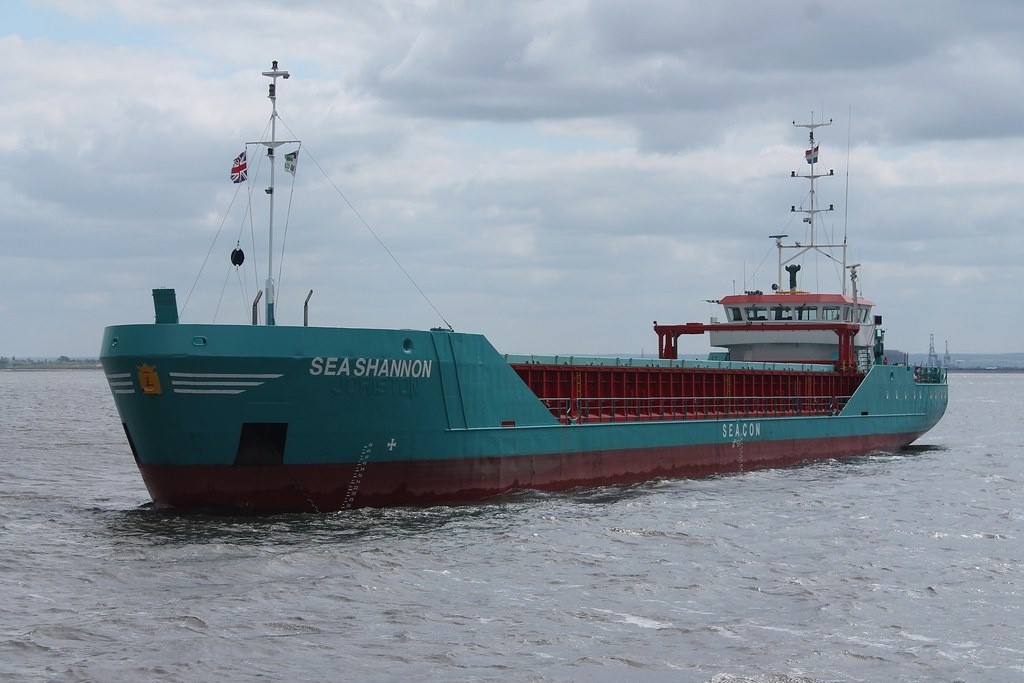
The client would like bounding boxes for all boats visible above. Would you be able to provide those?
[98,57,953,515]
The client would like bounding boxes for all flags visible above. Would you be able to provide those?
[805,146,818,164]
[284,150,298,175]
[231,151,247,184]
